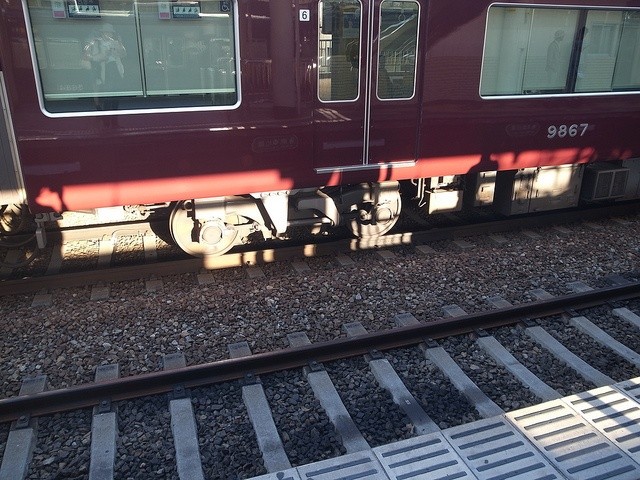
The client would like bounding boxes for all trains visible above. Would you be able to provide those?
[0,0,640,257]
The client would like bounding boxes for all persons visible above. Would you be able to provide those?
[570,27,593,87]
[218,47,234,69]
[85,24,126,87]
[547,29,563,87]
